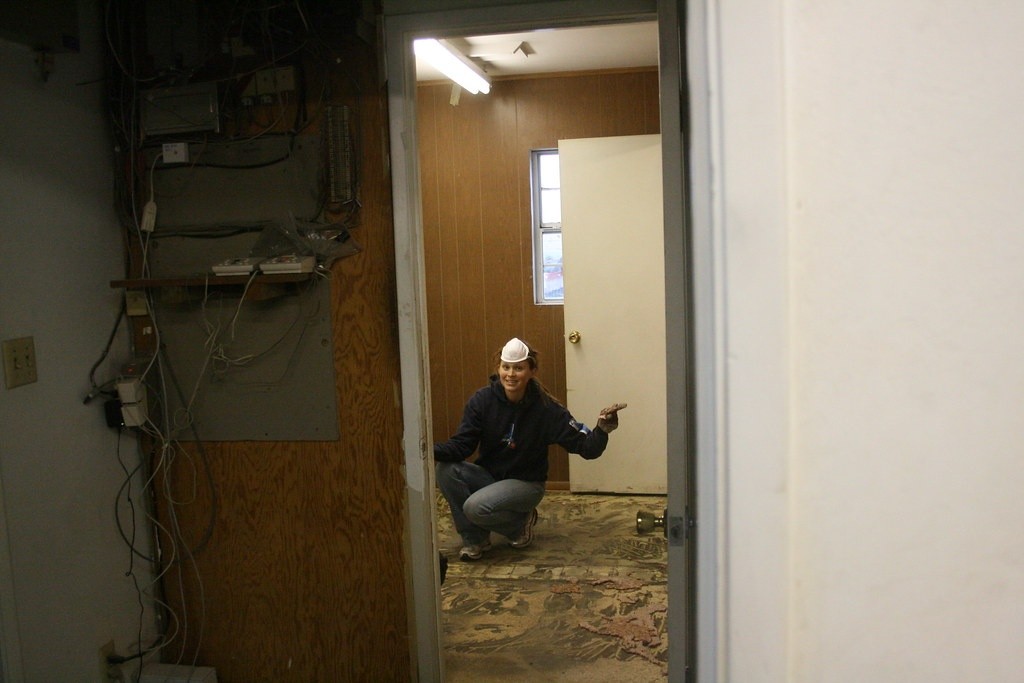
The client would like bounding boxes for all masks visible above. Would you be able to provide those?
[501,338,529,363]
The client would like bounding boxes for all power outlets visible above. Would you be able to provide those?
[98,641,118,683]
[255,66,295,96]
[1,336,39,391]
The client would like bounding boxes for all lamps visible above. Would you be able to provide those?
[414,39,492,95]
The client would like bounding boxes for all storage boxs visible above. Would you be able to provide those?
[137,663,217,683]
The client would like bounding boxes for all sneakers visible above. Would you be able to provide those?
[508,508,538,548]
[459,535,492,561]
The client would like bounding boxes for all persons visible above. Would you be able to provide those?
[433,337,628,562]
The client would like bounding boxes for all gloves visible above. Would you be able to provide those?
[597,403,627,433]
